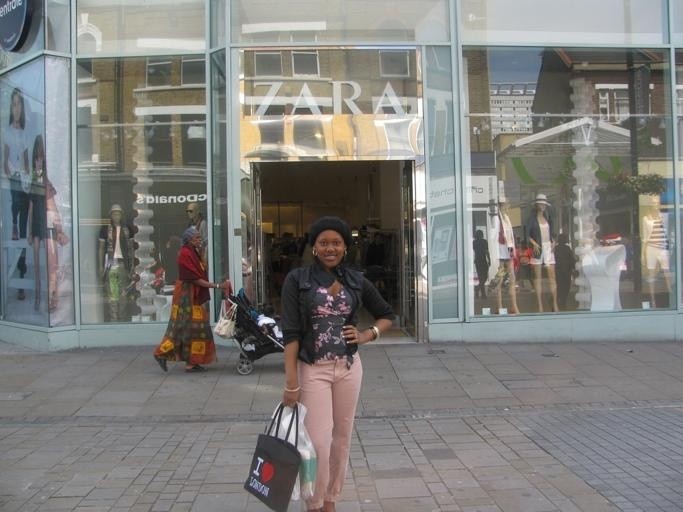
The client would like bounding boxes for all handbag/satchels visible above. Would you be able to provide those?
[214,298,239,340]
[243,399,319,512]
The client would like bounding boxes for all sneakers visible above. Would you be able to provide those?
[184,363,208,374]
[18,291,58,313]
[153,352,169,373]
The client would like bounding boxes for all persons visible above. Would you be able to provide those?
[27,134,70,315]
[280,214,396,512]
[525,192,561,312]
[640,196,670,307]
[97,203,136,322]
[3,86,30,301]
[486,204,520,315]
[166,203,208,267]
[152,225,227,373]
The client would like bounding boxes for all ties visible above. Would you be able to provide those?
[494,211,511,249]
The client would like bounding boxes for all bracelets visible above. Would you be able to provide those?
[368,324,381,344]
[217,283,220,289]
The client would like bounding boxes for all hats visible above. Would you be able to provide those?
[306,214,355,247]
[182,226,200,243]
[530,193,552,209]
[108,204,124,215]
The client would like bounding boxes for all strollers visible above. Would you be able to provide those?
[218,279,289,377]
